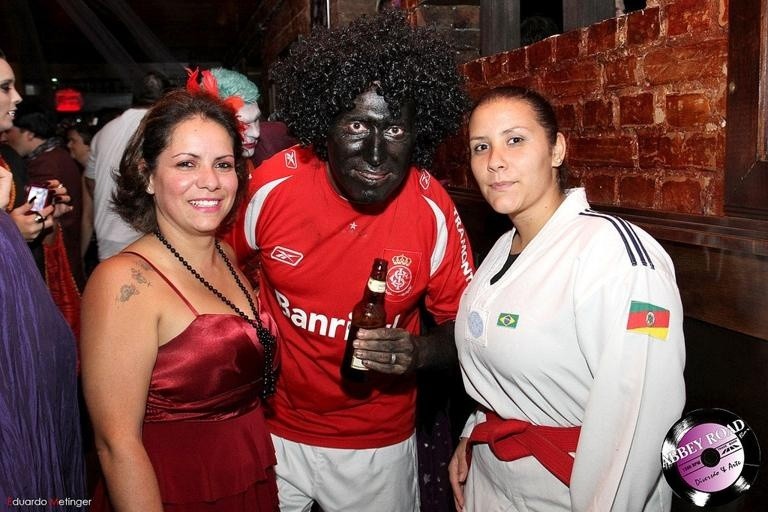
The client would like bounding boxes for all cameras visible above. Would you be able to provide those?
[23,179,53,215]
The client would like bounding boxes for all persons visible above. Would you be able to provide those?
[213,9,475,512]
[199,67,298,175]
[79,90,279,512]
[74,71,177,262]
[448,86,685,512]
[67,122,101,171]
[0,56,81,342]
[0,95,97,266]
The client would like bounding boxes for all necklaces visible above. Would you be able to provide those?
[154,226,278,399]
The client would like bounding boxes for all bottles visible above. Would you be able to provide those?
[340,258,389,381]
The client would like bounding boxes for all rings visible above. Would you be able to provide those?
[392,353,396,364]
[35,215,43,222]
